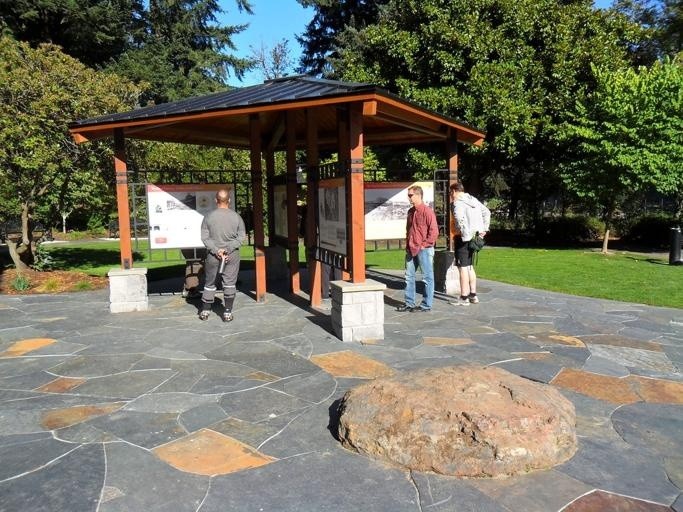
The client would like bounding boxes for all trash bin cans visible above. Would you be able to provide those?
[669,224,681,265]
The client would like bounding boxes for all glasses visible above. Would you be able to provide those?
[408,194,413,198]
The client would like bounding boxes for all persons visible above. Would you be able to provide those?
[198,190,246,322]
[296,197,316,278]
[398,186,439,313]
[447,183,491,307]
[180,249,207,298]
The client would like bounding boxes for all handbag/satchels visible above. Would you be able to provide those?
[469,232,485,253]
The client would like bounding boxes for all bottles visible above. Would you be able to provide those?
[217,251,229,275]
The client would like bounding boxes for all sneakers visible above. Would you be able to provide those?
[223,312,233,322]
[448,295,480,306]
[199,310,211,320]
[395,305,430,312]
